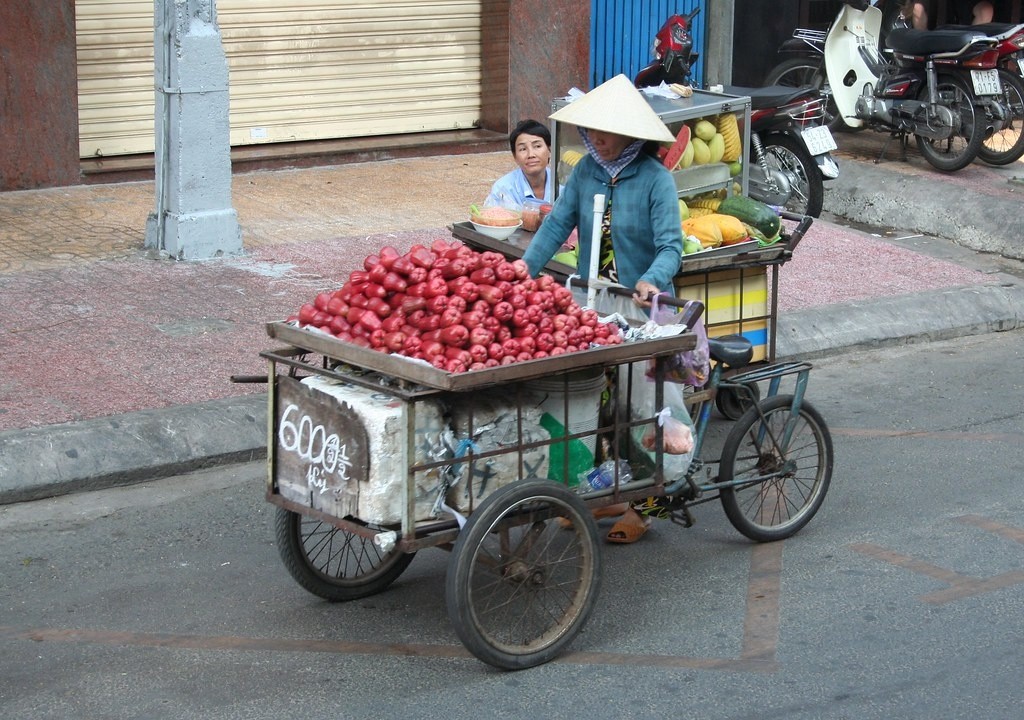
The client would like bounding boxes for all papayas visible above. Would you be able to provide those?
[682,213,749,248]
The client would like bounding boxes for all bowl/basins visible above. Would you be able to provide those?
[468,208,523,241]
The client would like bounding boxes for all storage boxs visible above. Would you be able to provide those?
[299,374,550,526]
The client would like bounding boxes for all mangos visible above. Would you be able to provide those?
[662,119,726,169]
[727,163,742,175]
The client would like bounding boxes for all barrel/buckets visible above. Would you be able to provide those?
[519,368,607,486]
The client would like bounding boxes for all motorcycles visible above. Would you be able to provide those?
[633,9,842,221]
[763,0,1024,173]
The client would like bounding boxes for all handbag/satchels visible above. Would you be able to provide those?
[566,276,710,482]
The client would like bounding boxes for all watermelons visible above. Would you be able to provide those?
[715,196,780,238]
[662,123,692,172]
[657,146,682,170]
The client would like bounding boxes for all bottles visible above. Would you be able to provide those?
[523,199,549,232]
[574,462,622,494]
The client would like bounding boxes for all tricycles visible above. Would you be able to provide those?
[259,274,835,673]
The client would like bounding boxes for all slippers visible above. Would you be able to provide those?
[559,503,627,528]
[606,521,651,543]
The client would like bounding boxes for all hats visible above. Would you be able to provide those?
[547,74,676,143]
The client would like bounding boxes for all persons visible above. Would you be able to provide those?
[906,0,994,31]
[520,74,682,543]
[484,119,566,208]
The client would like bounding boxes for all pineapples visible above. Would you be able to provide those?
[703,112,743,162]
[685,199,723,217]
[561,150,584,168]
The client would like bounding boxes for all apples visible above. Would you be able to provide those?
[682,234,705,256]
